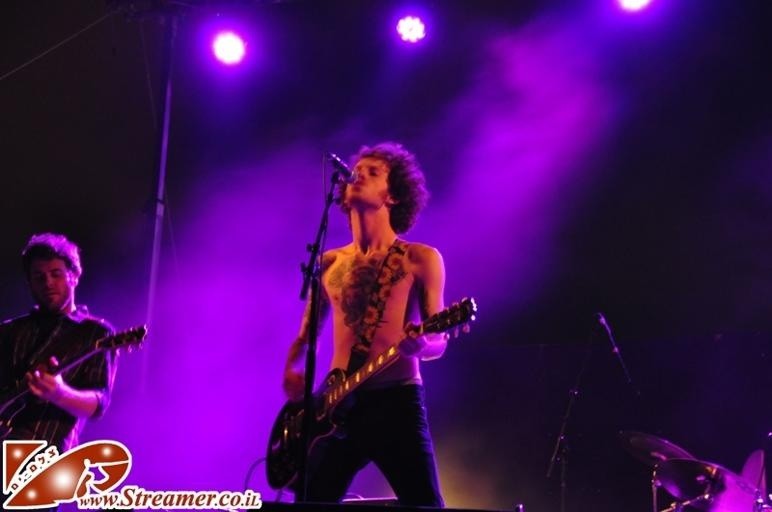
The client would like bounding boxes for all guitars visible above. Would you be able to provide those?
[1,324,147,440]
[266,296,478,492]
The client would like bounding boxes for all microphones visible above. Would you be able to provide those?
[327,153,358,184]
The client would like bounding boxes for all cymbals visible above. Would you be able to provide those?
[656,458,761,512]
[617,431,695,466]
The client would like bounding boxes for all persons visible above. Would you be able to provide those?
[1,230,122,457]
[279,140,448,510]
